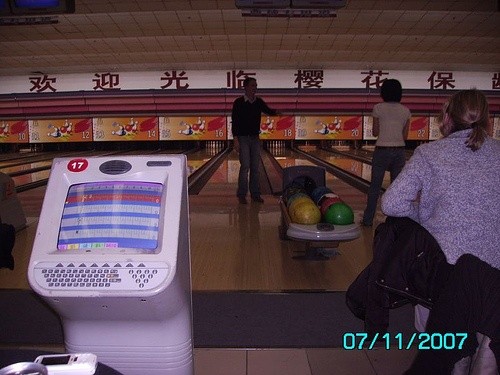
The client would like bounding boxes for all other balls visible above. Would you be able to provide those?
[285,184,354,224]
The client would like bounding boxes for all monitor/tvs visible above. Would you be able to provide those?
[0,0,75,16]
[57,181,162,249]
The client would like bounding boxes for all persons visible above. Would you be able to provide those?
[380,89,500,375]
[231,78,284,204]
[357,79,410,226]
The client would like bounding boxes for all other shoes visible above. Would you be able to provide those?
[250,195,265,202]
[239,196,249,204]
[357,213,371,226]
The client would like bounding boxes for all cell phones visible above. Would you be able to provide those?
[34,353,98,375]
